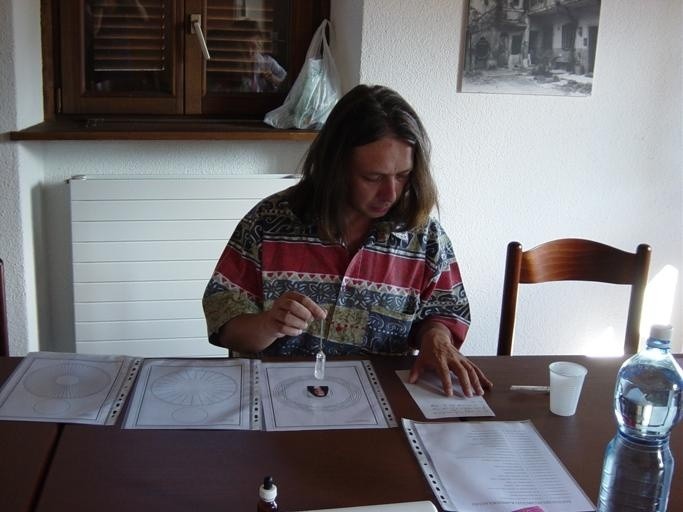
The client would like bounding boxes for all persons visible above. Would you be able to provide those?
[91,1,164,94]
[203,83,492,397]
[212,19,289,95]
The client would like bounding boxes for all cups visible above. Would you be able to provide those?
[548,361,588,417]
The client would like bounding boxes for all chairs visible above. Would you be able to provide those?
[496,238,652,356]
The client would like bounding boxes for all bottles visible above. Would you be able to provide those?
[596,325,682,512]
[256,475,277,512]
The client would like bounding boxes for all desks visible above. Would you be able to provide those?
[0,355,682,511]
